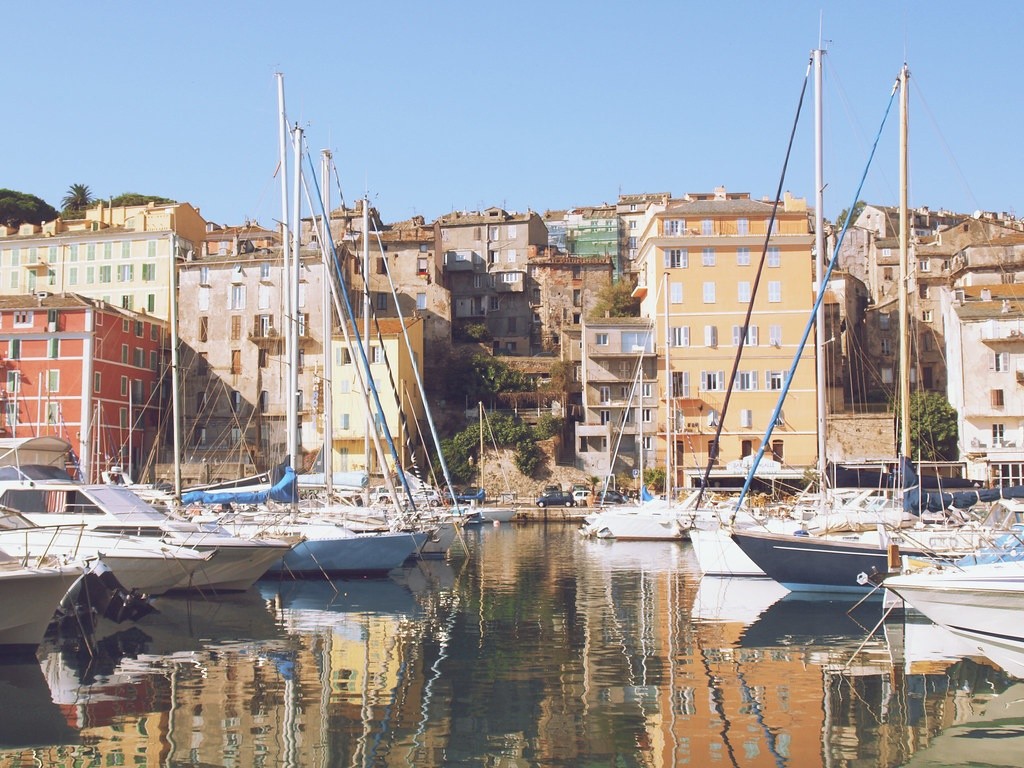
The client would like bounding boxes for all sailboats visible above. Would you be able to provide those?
[579,5,1024,682]
[99,66,521,574]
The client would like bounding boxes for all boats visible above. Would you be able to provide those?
[1,438,219,598]
[1,504,89,646]
[84,482,292,594]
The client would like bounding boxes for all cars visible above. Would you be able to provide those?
[571,491,592,506]
[536,491,574,507]
[459,486,486,503]
[442,486,462,504]
[401,490,443,507]
[596,491,629,504]
